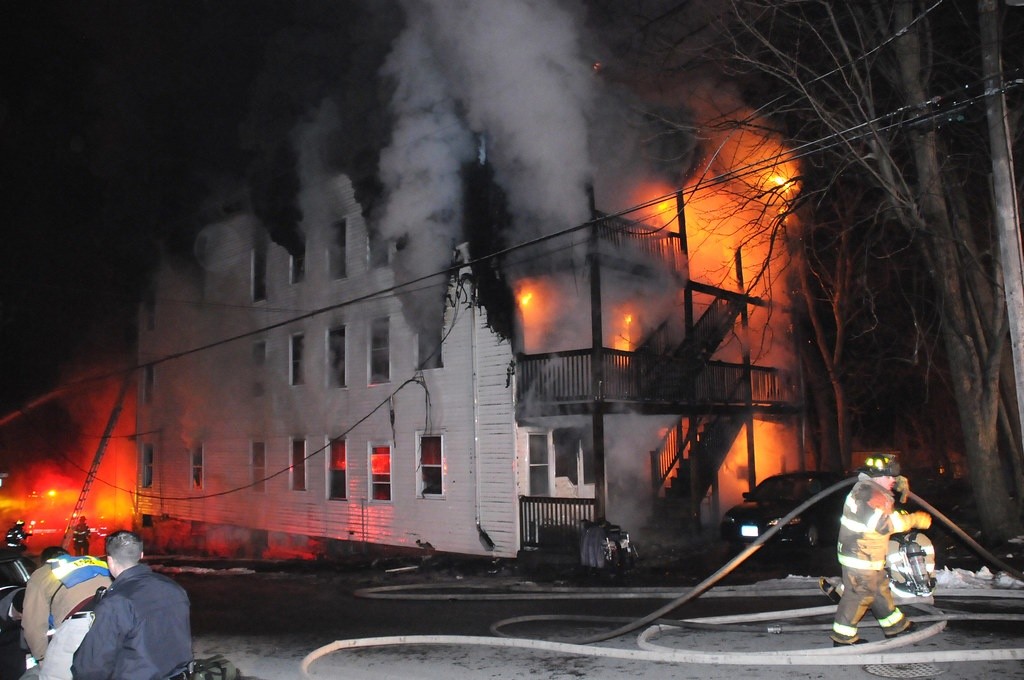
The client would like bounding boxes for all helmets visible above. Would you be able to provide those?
[857,454,900,478]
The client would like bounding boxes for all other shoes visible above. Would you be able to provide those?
[885,621,917,638]
[832,638,868,647]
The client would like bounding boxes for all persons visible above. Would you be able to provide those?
[578,516,616,587]
[0,516,191,680]
[818,452,936,648]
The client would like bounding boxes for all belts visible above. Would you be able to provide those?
[64,614,88,618]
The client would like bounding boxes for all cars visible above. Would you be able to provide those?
[0,547,38,679]
[719,471,855,550]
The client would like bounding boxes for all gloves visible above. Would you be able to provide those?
[914,511,931,530]
